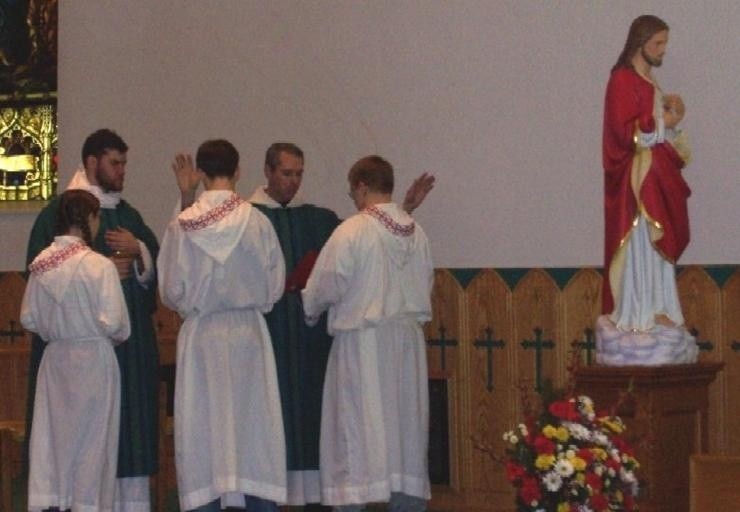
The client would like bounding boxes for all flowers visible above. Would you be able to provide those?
[467,331,643,512]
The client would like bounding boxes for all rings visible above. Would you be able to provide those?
[116,251,120,256]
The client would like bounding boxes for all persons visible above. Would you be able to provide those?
[19,189,130,511]
[169,142,439,510]
[22,127,159,508]
[155,137,287,511]
[300,152,434,512]
[601,15,692,338]
